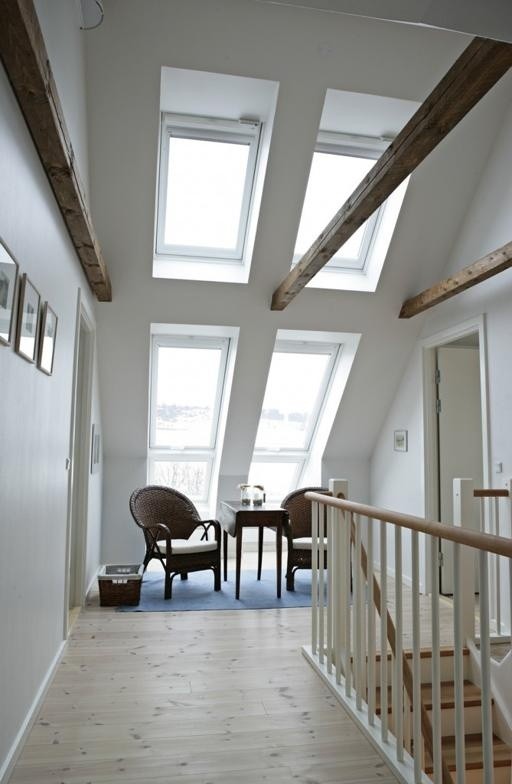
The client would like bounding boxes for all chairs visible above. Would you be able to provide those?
[129,485,221,600]
[281,487,357,595]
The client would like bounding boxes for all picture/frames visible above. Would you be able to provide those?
[14,273,41,364]
[36,301,58,376]
[0,238,19,345]
[394,430,408,453]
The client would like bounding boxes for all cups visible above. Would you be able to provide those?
[239,484,269,507]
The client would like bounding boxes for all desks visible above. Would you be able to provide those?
[219,500,287,600]
